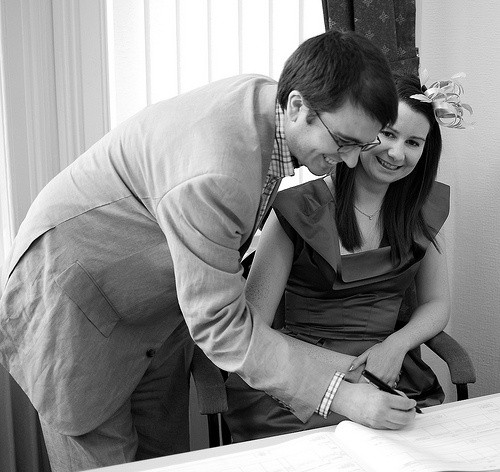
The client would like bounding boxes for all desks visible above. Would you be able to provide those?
[77,391,499,471]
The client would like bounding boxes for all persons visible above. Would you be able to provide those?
[219,71,453,445]
[0,29,418,472]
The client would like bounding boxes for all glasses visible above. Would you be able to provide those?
[310,102,381,152]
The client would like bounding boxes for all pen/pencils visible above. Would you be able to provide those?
[360,368,425,417]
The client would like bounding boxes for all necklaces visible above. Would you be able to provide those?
[354,204,382,220]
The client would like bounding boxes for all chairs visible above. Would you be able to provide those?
[207,246,478,448]
